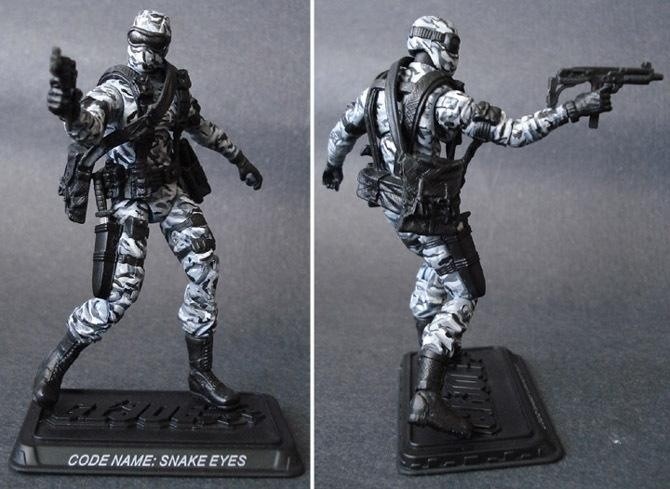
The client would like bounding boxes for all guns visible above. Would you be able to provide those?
[51,46,82,134]
[545,62,663,130]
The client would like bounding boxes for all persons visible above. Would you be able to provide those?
[318,12,614,443]
[26,8,267,413]
